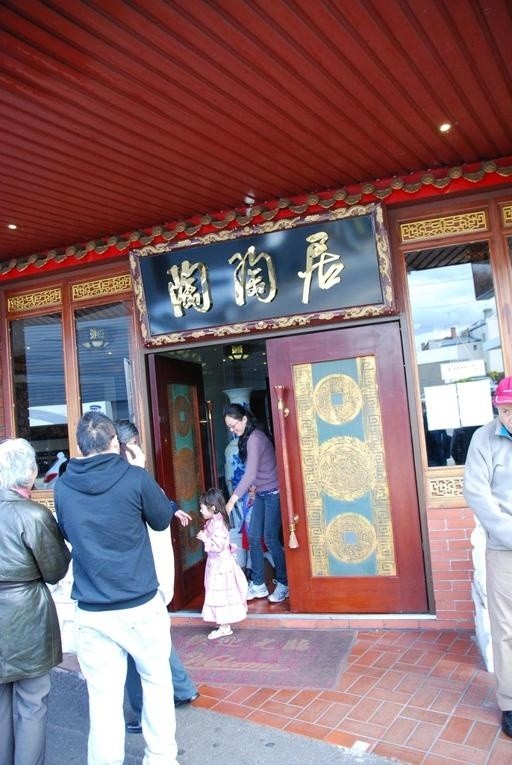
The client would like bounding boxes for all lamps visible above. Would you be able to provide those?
[224,343,251,363]
[80,328,112,351]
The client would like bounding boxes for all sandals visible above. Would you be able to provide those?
[207,625,234,640]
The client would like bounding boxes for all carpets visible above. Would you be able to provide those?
[171,625,357,692]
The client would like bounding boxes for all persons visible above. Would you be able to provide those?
[222,404,288,602]
[53,412,199,765]
[193,488,248,640]
[463,376,512,737]
[0,438,70,765]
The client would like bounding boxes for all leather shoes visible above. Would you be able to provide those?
[126,721,142,734]
[174,692,201,708]
[502,710,512,738]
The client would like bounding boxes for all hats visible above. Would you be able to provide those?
[494,377,512,405]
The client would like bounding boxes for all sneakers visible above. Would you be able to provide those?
[247,581,269,601]
[267,583,288,603]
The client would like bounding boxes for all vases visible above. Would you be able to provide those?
[221,387,255,530]
